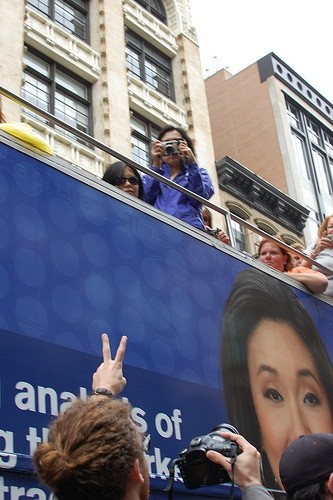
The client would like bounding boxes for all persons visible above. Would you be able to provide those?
[200,205,232,245]
[279,433,333,500]
[222,270,333,491]
[102,161,145,201]
[142,125,215,232]
[36,332,276,500]
[252,214,333,293]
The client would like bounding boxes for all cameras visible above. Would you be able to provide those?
[179,423,243,490]
[159,140,180,156]
[208,229,218,236]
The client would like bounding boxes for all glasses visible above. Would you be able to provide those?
[114,176,140,185]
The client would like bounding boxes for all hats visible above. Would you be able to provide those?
[278,434,333,493]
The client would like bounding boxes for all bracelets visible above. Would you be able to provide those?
[91,388,116,399]
